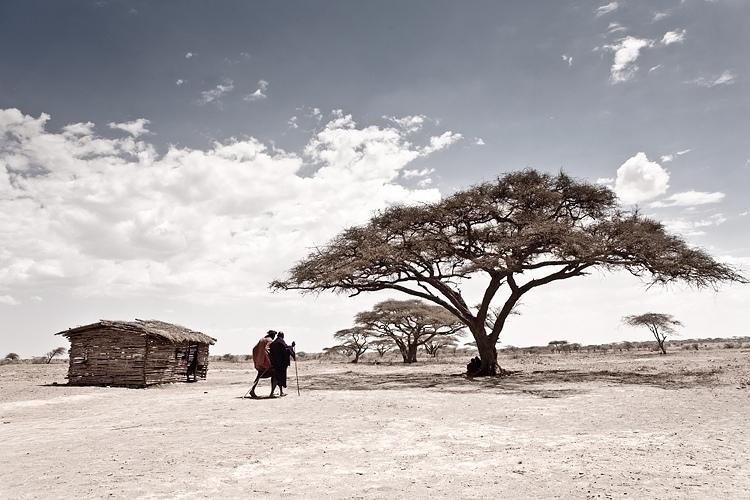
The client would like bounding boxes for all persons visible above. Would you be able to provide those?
[269,332,295,398]
[249,330,277,398]
